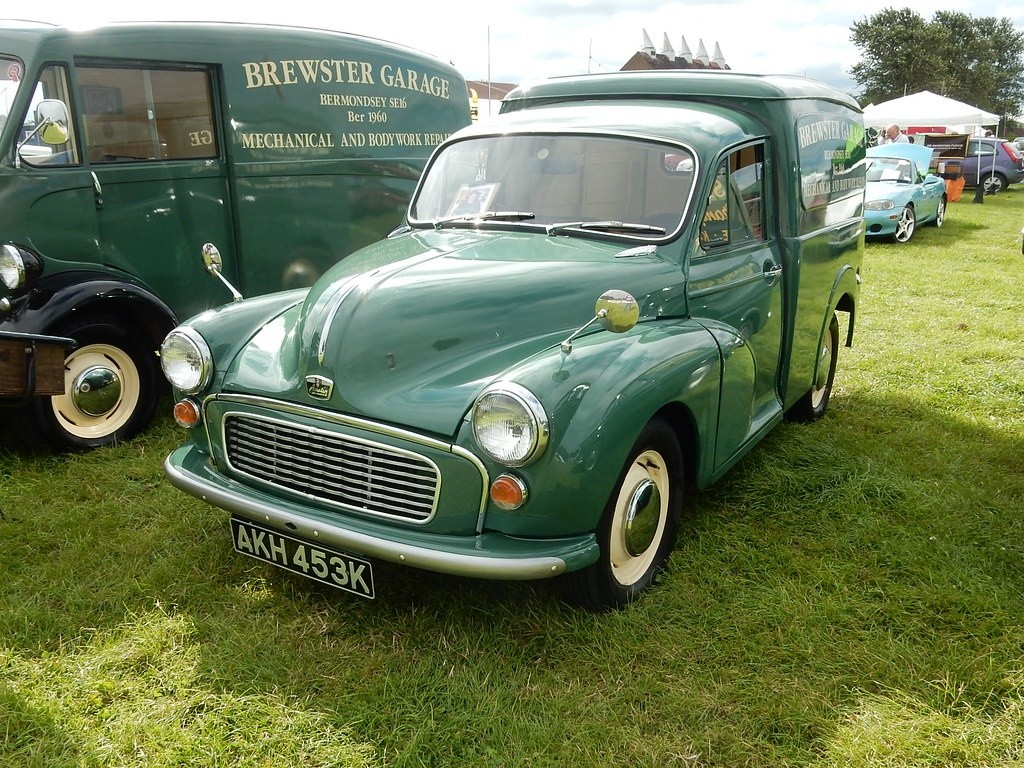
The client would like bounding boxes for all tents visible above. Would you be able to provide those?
[863,91,999,203]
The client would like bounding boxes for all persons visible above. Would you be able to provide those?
[981,131,994,152]
[886,125,908,143]
[908,137,914,143]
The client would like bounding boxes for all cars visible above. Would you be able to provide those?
[931,137,1024,193]
[863,144,948,244]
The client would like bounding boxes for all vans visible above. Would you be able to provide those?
[0,21,477,448]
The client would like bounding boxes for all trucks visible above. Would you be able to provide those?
[160,68,869,605]
